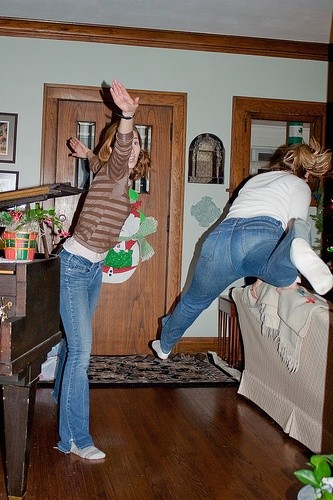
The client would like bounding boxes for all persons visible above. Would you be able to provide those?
[51,80,156,460]
[151,136,333,360]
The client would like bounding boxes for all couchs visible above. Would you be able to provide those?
[231,277,333,457]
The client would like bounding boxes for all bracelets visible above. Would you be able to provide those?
[114,112,135,120]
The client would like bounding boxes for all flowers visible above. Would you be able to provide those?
[292,454,333,500]
[0,206,68,236]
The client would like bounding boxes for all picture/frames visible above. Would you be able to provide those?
[0,170,19,227]
[0,112,19,163]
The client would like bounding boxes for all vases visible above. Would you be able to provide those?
[4,229,39,261]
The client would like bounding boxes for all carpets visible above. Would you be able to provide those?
[39,349,242,388]
